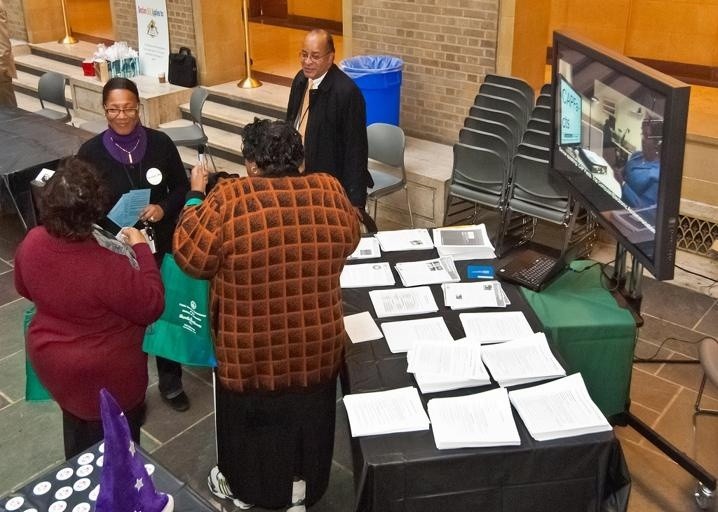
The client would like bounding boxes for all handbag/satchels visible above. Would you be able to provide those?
[168,47,196,87]
[142,253,217,368]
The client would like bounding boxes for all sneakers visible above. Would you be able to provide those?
[169,392,189,411]
[208,465,255,510]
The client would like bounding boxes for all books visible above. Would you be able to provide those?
[467,264,495,279]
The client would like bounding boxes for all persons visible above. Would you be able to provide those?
[287,26,377,232]
[172,116,361,512]
[11,157,167,463]
[613,115,659,230]
[73,76,193,414]
[0,0,20,109]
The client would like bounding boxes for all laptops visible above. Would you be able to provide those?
[495,227,597,292]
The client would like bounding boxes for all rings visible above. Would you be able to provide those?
[150,216,155,221]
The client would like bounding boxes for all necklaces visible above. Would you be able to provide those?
[107,133,144,165]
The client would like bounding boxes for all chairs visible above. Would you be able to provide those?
[442,74,602,268]
[32,72,76,128]
[153,87,217,174]
[362,123,415,235]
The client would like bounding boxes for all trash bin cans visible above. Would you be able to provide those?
[338,54,404,128]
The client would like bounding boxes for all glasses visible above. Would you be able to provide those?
[300,52,329,61]
[104,105,138,115]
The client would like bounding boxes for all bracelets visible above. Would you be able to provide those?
[620,180,627,188]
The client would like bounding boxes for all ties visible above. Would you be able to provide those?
[298,80,314,173]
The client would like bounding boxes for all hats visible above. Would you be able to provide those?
[95,388,174,512]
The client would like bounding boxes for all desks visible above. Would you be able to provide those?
[0,103,99,233]
[338,222,615,496]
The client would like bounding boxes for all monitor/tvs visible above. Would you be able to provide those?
[548,27,693,282]
